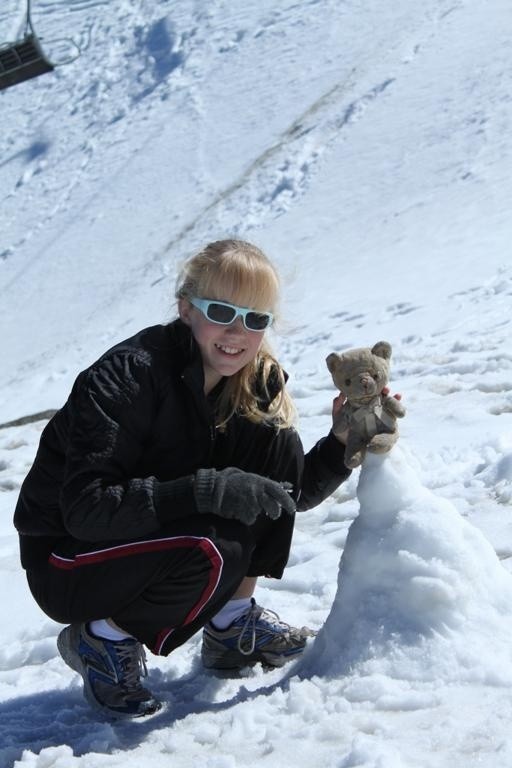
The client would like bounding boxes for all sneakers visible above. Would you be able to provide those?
[199,595,325,671]
[56,618,163,718]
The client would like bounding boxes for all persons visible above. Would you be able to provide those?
[13,237,406,719]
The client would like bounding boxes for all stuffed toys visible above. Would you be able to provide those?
[323,339,406,472]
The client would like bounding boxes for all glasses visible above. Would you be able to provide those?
[189,296,274,332]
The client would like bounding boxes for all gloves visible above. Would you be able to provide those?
[195,465,298,524]
